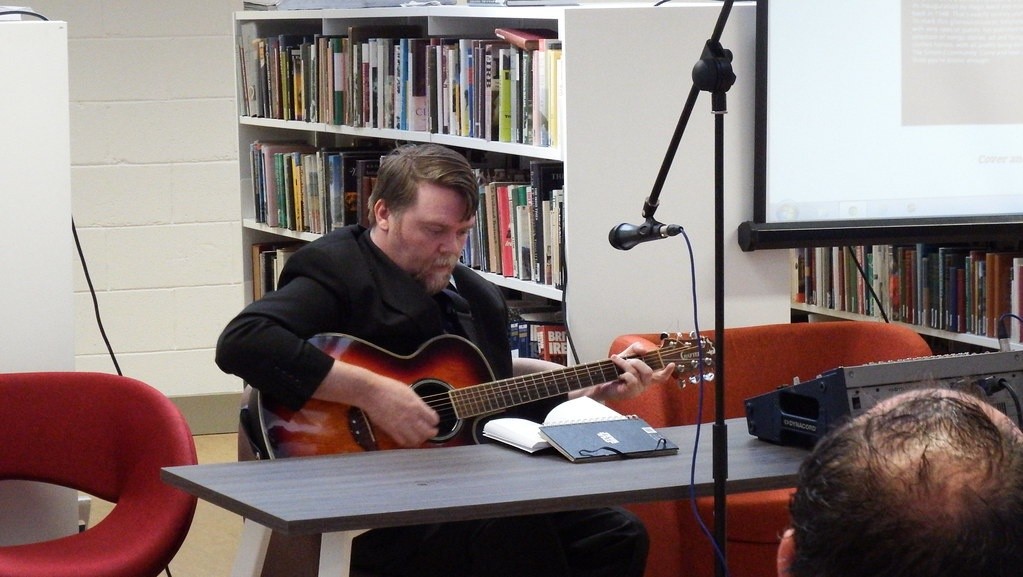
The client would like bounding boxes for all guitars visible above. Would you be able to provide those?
[247,331,718,460]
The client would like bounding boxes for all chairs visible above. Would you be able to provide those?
[0,370,198,577]
[231,356,605,577]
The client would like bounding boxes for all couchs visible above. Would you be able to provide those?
[606,321,934,577]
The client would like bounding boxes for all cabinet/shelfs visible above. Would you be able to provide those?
[789,244,1023,353]
[227,0,784,368]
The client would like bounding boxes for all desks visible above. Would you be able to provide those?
[160,418,815,577]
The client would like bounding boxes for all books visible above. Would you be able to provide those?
[795,243,1023,344]
[483,395,679,464]
[238,27,568,367]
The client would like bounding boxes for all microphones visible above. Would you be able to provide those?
[609,223,684,250]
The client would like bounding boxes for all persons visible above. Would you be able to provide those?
[777,389,1023,577]
[214,144,675,577]
